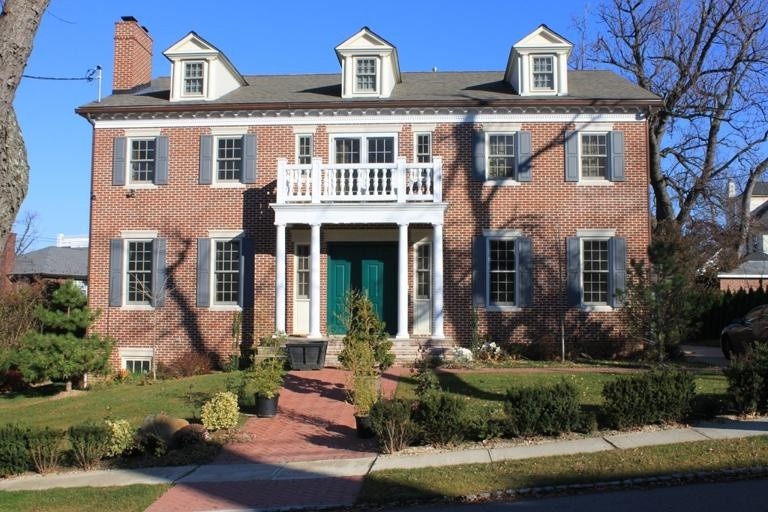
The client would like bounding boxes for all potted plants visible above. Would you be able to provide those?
[337,287,397,406]
[345,376,382,434]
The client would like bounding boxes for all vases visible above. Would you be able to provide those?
[250,331,288,418]
